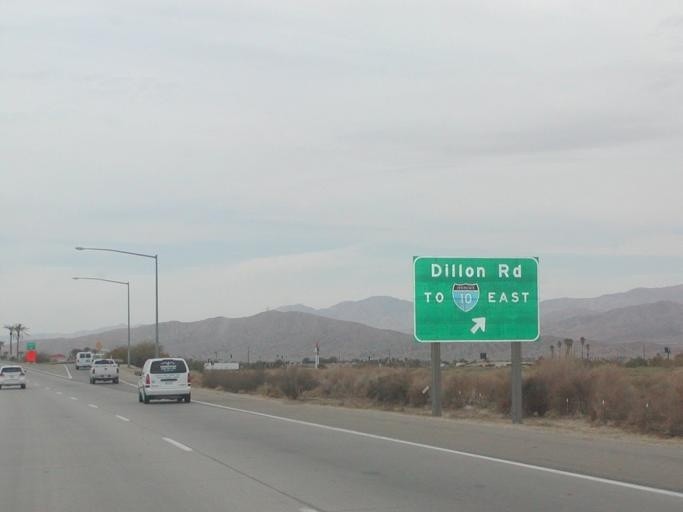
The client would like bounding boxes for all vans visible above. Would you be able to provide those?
[138,358,193,404]
[75,351,92,370]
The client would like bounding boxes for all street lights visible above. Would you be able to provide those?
[75,247,159,357]
[74,278,130,366]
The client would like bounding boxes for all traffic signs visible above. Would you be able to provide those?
[411,256,541,344]
[26,342,37,350]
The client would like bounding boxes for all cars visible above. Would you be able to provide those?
[0,366,26,388]
[90,358,119,383]
